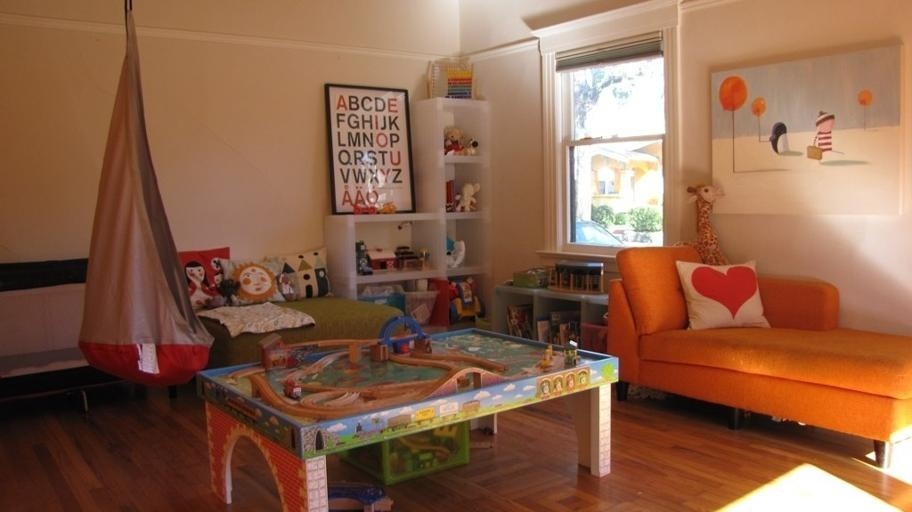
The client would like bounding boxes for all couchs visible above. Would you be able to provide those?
[606,246,912,468]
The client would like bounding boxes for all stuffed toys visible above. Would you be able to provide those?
[670,185,730,265]
[446,237,466,267]
[454,183,480,212]
[467,136,481,155]
[445,127,469,156]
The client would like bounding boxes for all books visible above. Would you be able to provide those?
[505,302,581,348]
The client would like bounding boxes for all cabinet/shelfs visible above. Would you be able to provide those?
[491,284,608,357]
[322,97,491,333]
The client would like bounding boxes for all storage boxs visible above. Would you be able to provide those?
[357,284,440,327]
[338,420,470,486]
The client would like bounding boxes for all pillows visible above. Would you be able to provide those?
[674,259,772,330]
[176,247,335,313]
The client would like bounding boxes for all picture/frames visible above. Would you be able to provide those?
[326,82,416,213]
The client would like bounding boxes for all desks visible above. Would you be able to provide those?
[196,327,619,512]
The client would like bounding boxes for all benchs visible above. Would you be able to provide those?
[1,259,135,422]
[195,296,406,370]
